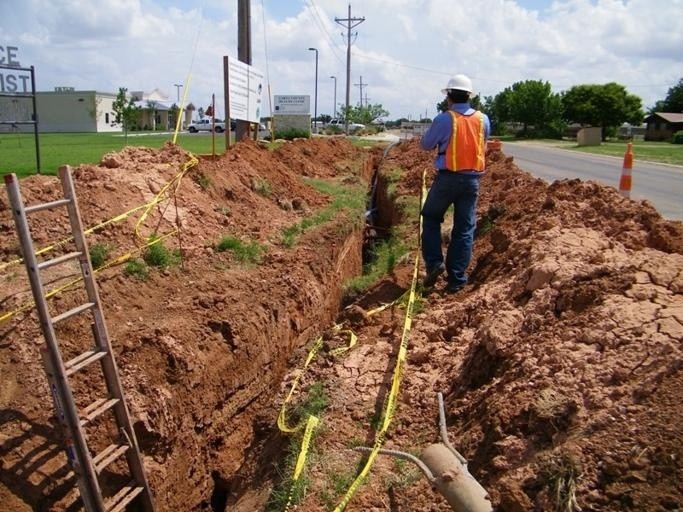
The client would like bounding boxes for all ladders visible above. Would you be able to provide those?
[3,164,156,512]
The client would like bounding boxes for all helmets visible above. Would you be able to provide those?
[440,74,473,95]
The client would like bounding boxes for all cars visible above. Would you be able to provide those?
[328,118,366,131]
[224,118,236,130]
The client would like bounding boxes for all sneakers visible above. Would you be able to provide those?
[423,263,465,291]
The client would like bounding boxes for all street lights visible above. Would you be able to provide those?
[309,48,318,128]
[174,84,183,102]
[330,77,336,118]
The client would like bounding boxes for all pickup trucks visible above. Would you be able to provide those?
[187,117,226,133]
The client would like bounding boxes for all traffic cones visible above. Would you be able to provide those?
[618,142,634,199]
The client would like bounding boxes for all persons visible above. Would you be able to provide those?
[419,73,492,295]
